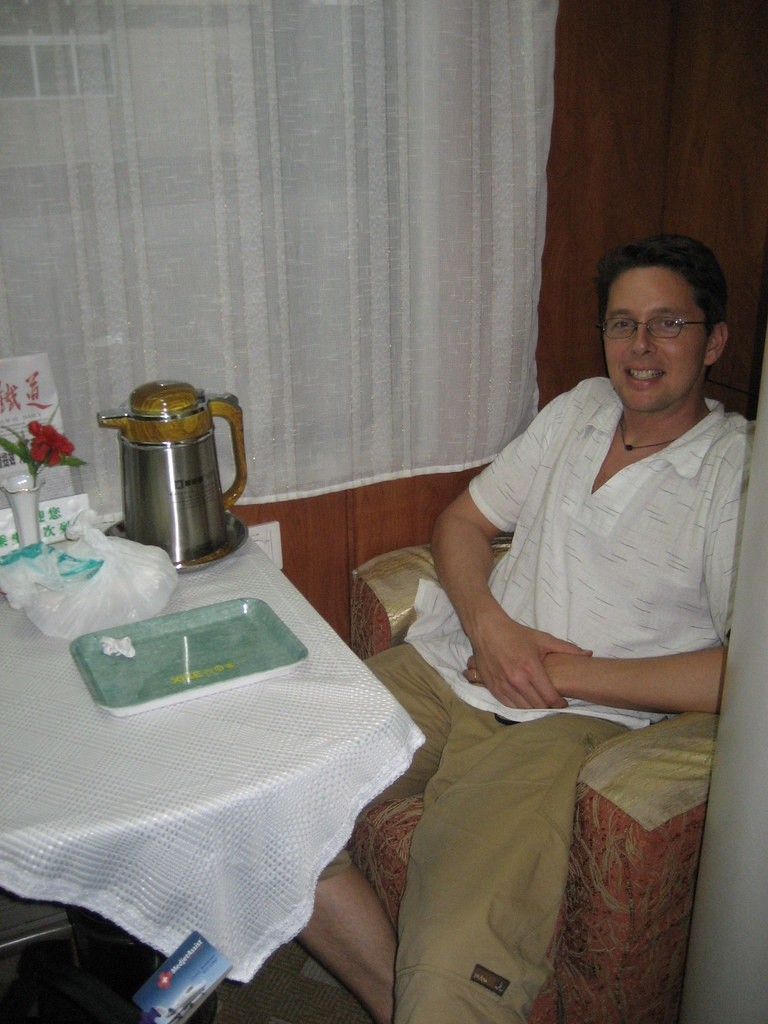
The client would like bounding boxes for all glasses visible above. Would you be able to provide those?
[596,316,707,338]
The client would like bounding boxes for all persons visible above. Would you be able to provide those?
[300,237,755,1024]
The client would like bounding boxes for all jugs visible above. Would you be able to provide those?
[98,381,247,563]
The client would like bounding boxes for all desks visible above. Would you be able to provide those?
[0,508,427,986]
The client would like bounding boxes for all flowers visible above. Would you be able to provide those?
[0,395,90,490]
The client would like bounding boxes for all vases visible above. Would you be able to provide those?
[0,474,46,550]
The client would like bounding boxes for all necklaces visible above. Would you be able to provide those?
[618,415,678,452]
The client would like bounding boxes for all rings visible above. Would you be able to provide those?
[473,669,477,682]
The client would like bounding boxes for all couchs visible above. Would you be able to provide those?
[348,533,720,1024]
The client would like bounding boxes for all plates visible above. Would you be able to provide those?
[69,597,308,717]
[105,512,249,574]
